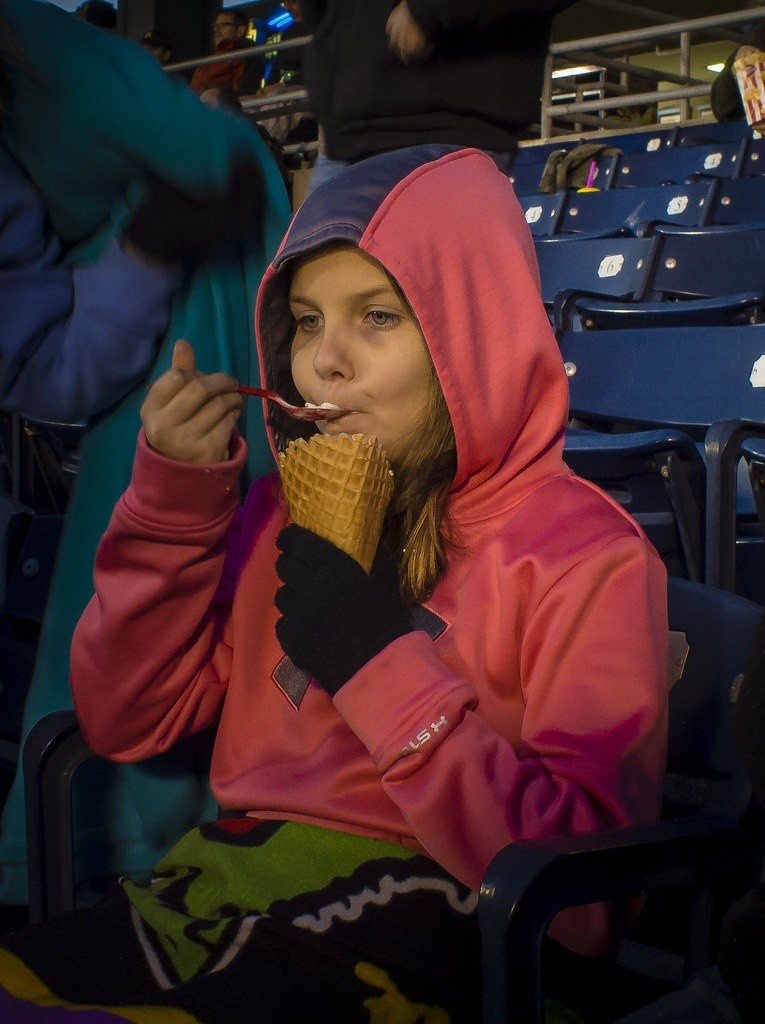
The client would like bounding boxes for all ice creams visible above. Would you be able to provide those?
[276,432,394,577]
[304,402,342,410]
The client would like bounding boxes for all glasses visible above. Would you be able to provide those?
[212,22,236,28]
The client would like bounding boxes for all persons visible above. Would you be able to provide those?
[75,0,309,172]
[0,140,667,1024]
[302,0,580,200]
[1,142,267,431]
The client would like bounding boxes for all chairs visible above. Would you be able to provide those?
[1,121,765,1024]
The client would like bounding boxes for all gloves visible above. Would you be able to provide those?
[276,524,401,695]
[120,148,269,282]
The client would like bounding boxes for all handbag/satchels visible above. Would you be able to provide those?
[116,818,479,1023]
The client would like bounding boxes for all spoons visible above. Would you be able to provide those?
[147,384,350,422]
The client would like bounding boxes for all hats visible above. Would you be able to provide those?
[136,31,176,50]
